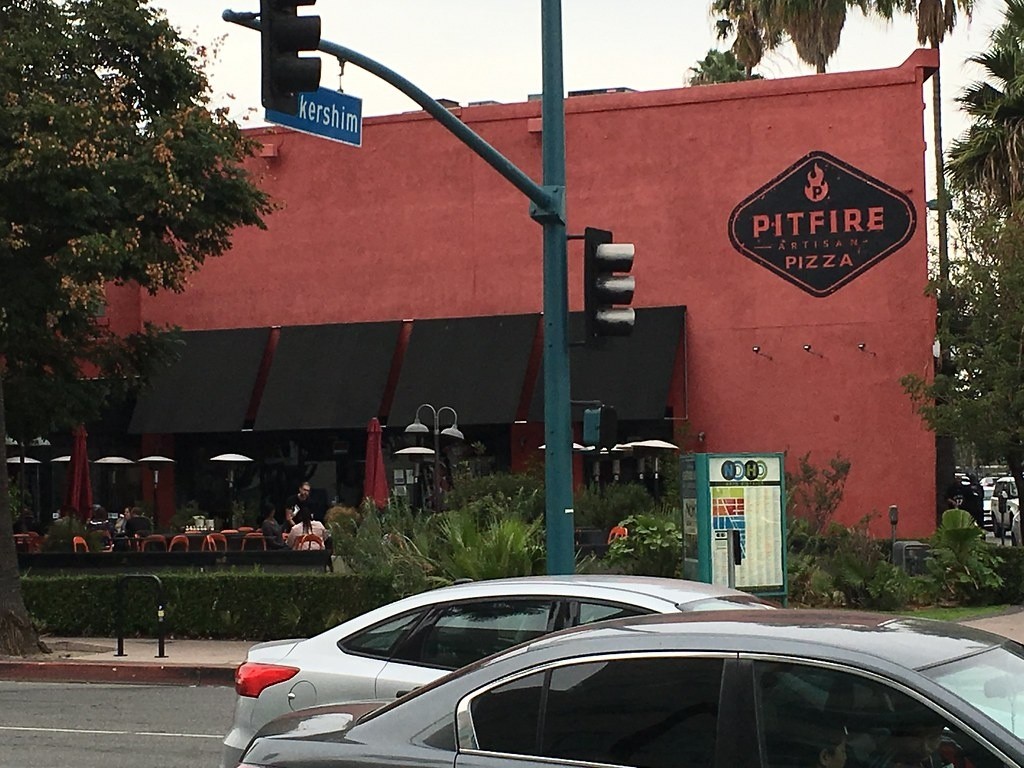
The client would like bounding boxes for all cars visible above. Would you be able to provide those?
[232,608,1024,768]
[220,573,779,768]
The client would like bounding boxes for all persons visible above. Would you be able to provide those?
[264,478,328,554]
[88,503,154,553]
[867,701,959,768]
[788,716,849,768]
[955,472,986,532]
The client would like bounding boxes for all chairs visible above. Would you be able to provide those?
[206,535,218,550]
[12,534,31,554]
[72,535,88,553]
[201,532,227,552]
[168,534,190,553]
[290,535,302,550]
[240,532,266,550]
[27,531,42,552]
[607,525,628,547]
[139,534,169,553]
[125,533,141,549]
[84,528,113,552]
[298,534,322,551]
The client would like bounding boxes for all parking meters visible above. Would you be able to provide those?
[725,527,743,588]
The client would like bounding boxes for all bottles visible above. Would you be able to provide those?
[184,525,215,532]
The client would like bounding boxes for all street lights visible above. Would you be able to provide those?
[405,403,464,511]
